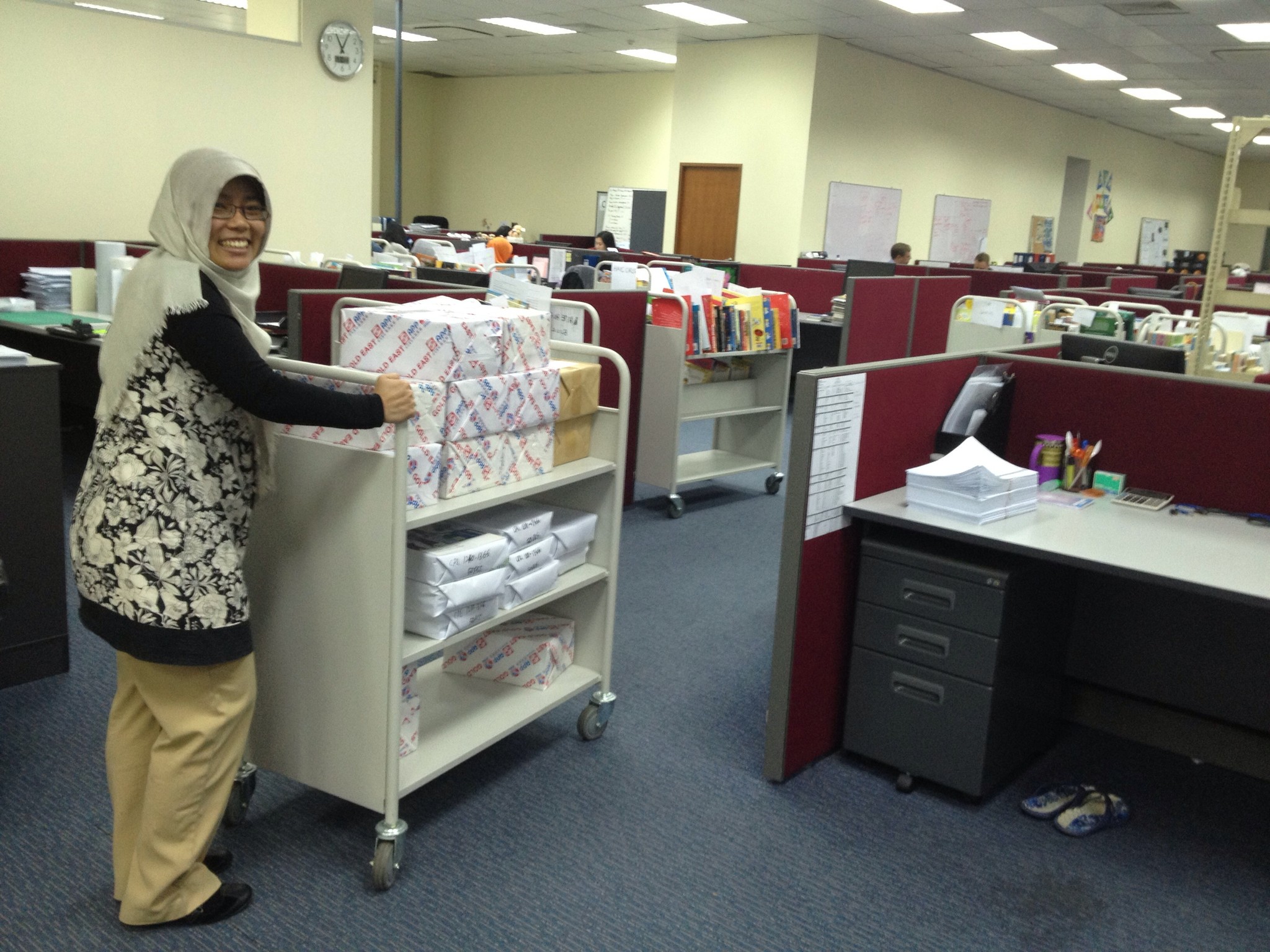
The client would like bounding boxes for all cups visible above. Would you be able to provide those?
[1062,454,1091,493]
[1028,434,1066,485]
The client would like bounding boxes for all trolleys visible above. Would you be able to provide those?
[592,258,796,519]
[946,293,1253,358]
[219,294,634,895]
[259,236,542,290]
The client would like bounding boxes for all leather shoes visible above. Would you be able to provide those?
[202,851,230,875]
[124,882,252,932]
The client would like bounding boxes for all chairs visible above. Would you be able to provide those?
[414,216,448,229]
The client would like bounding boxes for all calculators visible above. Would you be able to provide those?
[1112,485,1176,511]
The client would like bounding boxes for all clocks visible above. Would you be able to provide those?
[319,19,365,79]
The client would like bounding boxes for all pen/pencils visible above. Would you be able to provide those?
[1064,431,1103,491]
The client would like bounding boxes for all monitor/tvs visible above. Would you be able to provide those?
[1060,333,1186,373]
[535,240,573,247]
[1024,262,1063,275]
[415,267,490,287]
[336,263,390,289]
[1128,286,1184,298]
[842,259,897,296]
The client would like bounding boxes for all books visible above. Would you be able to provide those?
[1148,331,1260,369]
[831,294,846,325]
[637,267,800,355]
[683,358,752,385]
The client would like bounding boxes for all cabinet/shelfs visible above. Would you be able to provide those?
[1173,249,1209,270]
[843,536,1079,801]
[1,355,71,689]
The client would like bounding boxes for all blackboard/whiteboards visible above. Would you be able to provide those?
[929,194,992,264]
[1135,217,1170,268]
[823,181,903,263]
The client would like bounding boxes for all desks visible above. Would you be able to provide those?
[0,308,114,346]
[843,487,1270,612]
[800,312,843,328]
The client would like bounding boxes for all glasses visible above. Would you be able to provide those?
[212,202,269,220]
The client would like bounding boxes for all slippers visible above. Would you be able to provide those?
[1056,793,1131,837]
[1020,784,1095,819]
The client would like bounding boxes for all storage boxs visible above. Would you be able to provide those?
[441,611,576,690]
[399,661,421,756]
[270,296,601,509]
[406,504,599,643]
[1092,470,1127,496]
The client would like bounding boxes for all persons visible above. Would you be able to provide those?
[967,253,992,270]
[69,147,415,925]
[595,231,615,251]
[488,225,513,270]
[888,243,912,264]
